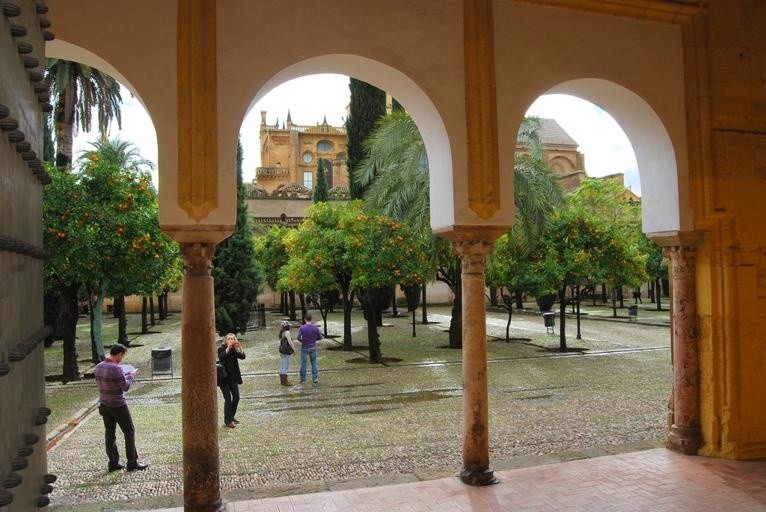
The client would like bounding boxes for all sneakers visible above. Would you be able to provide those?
[300,379,318,384]
[226,419,240,427]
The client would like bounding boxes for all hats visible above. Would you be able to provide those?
[281,321,292,328]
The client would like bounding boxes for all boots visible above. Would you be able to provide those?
[279,374,292,386]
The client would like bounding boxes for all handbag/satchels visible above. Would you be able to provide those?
[279,336,293,354]
[216,361,228,380]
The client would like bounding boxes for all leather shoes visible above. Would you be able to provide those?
[128,463,148,471]
[109,464,125,472]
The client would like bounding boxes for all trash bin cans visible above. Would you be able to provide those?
[628,305,637,321]
[151,347,172,383]
[543,313,555,334]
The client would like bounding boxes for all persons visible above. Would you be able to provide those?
[277,320,296,386]
[92,343,149,472]
[297,313,323,383]
[217,332,246,428]
[305,294,332,314]
